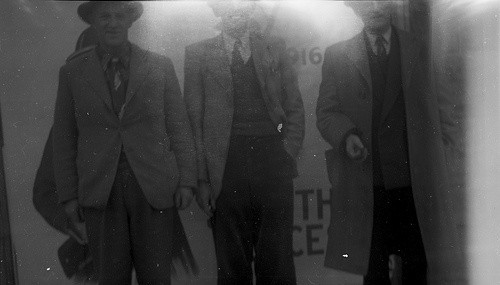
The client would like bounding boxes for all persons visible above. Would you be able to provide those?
[181,2,308,285]
[316,0,447,284]
[29,1,197,284]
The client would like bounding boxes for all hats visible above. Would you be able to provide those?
[77,1,144,21]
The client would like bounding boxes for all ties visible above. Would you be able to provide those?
[374,35,389,82]
[109,56,126,116]
[231,40,245,75]
